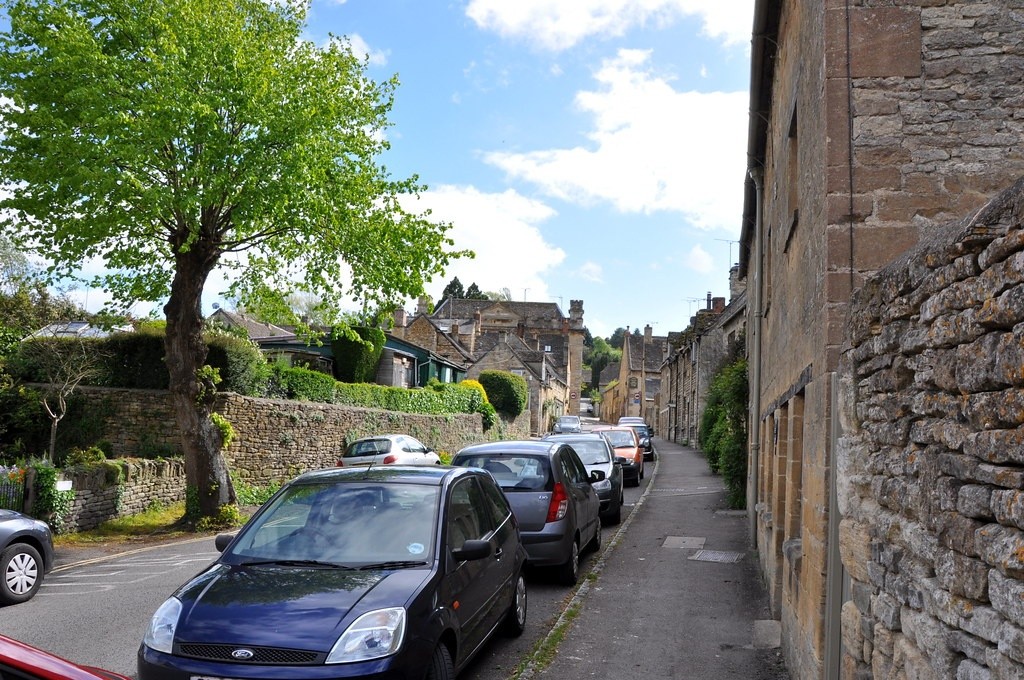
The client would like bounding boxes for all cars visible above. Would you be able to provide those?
[451,440,606,587]
[552,415,585,434]
[586,403,594,414]
[615,415,655,461]
[0,508,57,606]
[135,465,532,680]
[335,434,441,472]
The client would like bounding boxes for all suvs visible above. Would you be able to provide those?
[589,425,646,487]
[513,430,627,525]
